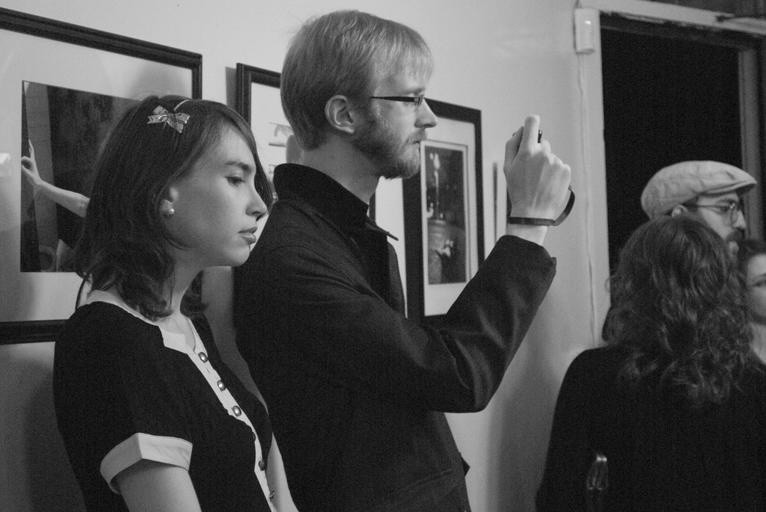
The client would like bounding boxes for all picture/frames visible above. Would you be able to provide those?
[235,62,295,323]
[406,98,483,325]
[0,6,205,345]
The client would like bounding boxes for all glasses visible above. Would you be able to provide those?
[682,202,743,225]
[370,93,424,112]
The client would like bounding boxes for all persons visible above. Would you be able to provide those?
[641,159,766,373]
[229,10,574,512]
[50,93,298,512]
[734,241,766,369]
[528,214,766,512]
[21,134,99,269]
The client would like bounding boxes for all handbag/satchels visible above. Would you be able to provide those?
[578,444,610,512]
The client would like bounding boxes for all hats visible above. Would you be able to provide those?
[641,160,758,220]
